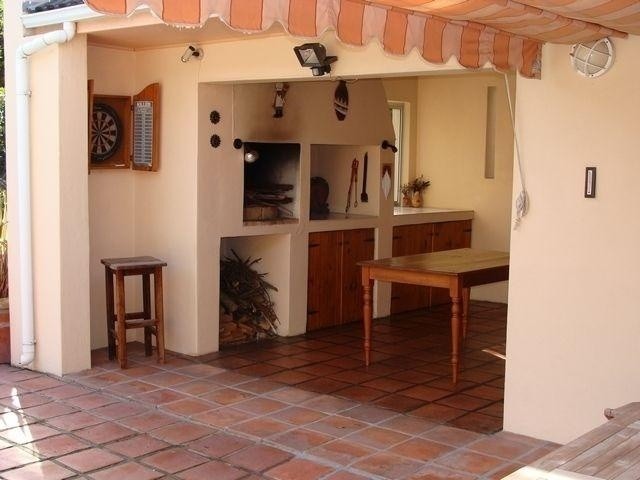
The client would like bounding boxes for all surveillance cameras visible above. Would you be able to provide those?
[181,45,196,63]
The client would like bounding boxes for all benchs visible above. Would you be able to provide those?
[500,402,640,480]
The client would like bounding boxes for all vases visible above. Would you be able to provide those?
[402,194,411,207]
[412,192,421,207]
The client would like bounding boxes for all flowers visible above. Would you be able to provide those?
[410,174,431,192]
[398,183,412,195]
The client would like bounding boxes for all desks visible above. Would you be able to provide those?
[355,247,509,383]
[101,255,170,368]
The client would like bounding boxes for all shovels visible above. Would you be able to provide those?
[361,152,368,202]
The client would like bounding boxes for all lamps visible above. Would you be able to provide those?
[571,38,615,78]
[295,42,338,78]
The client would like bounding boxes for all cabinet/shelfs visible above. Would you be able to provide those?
[87,79,161,174]
